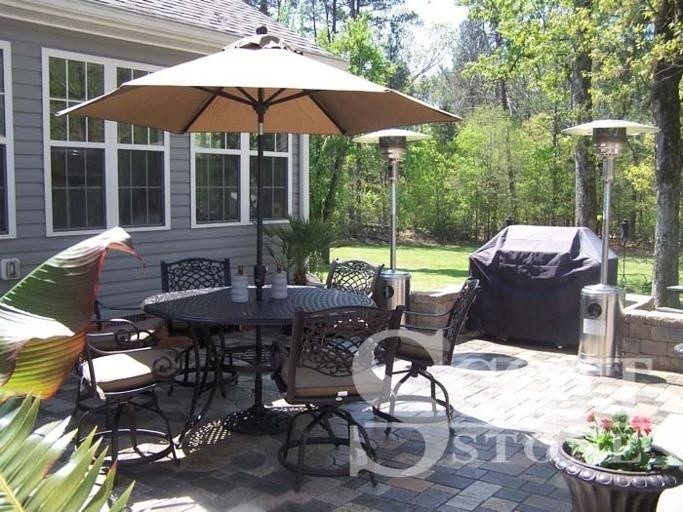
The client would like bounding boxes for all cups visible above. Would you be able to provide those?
[270,271,288,300]
[231,273,248,304]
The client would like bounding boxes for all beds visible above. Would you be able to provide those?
[142,284,380,437]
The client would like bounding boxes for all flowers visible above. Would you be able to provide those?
[567,406,683,471]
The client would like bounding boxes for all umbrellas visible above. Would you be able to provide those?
[52,26,465,408]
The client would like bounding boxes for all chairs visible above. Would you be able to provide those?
[270,303,405,493]
[159,256,240,398]
[325,257,387,291]
[73,296,193,484]
[373,278,484,439]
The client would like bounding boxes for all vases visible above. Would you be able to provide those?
[546,434,683,512]
